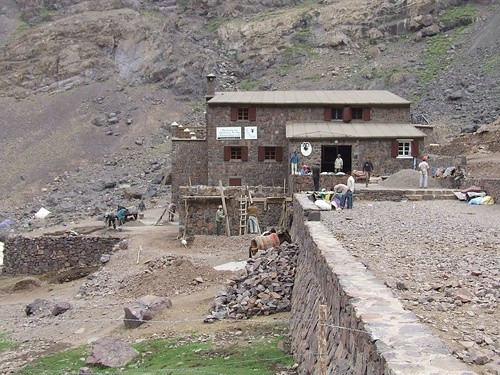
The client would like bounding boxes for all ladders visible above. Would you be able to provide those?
[238,195,249,235]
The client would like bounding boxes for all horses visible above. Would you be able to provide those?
[249,229,292,258]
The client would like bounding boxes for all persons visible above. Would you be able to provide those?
[310,161,320,191]
[107,212,116,230]
[418,156,430,188]
[215,204,228,236]
[333,154,343,174]
[167,202,176,222]
[331,171,355,211]
[289,150,300,176]
[263,228,276,236]
[362,156,374,187]
[116,208,129,226]
[247,202,259,234]
